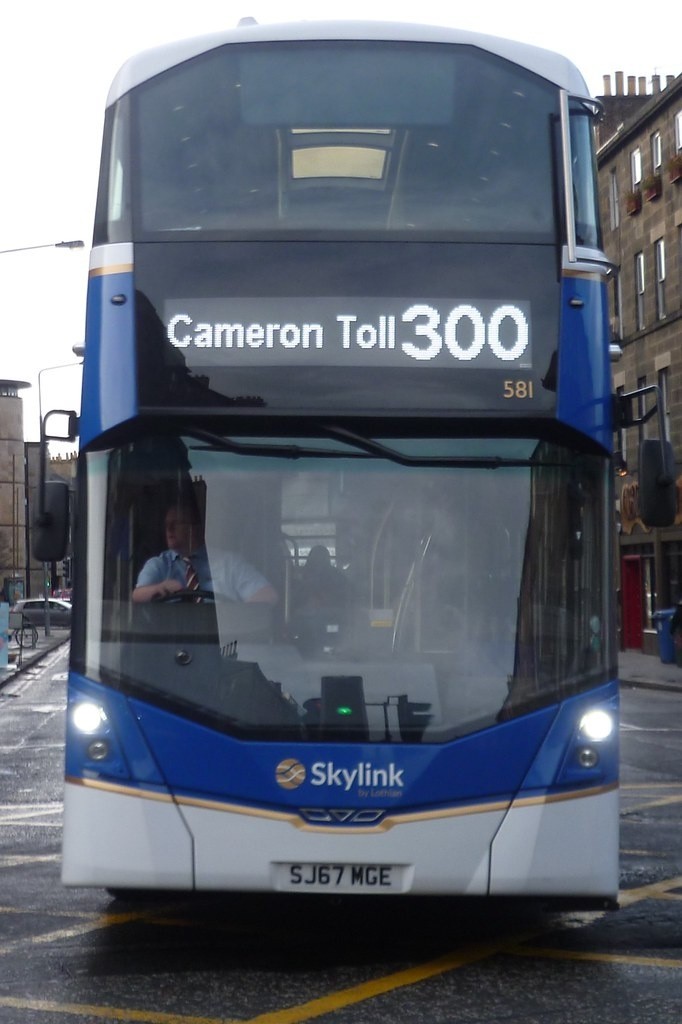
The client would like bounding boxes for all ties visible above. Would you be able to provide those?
[180,555,203,605]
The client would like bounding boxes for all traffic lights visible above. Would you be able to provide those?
[29,16,682,920]
[46,575,50,588]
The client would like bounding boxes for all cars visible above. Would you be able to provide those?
[12,598,72,626]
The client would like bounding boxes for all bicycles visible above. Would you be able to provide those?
[8,615,38,648]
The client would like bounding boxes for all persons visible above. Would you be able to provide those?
[300,545,353,600]
[132,503,279,606]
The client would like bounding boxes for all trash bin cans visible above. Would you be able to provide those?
[653,608,679,665]
